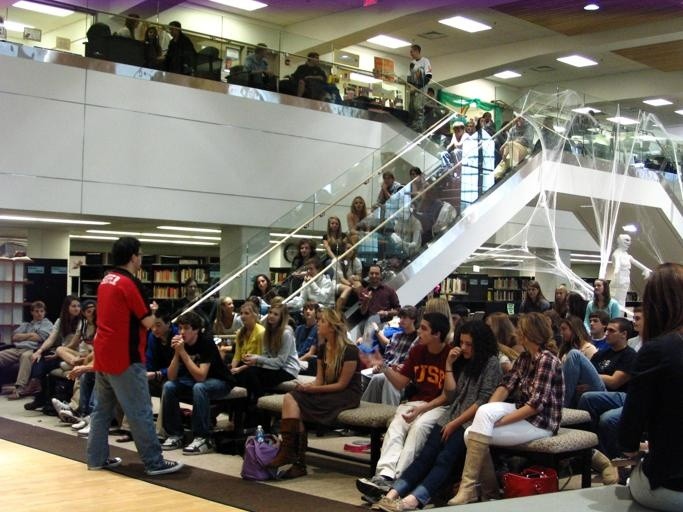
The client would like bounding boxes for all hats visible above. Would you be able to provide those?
[80,299,95,311]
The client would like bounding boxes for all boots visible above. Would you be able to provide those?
[478,447,499,503]
[267,417,300,468]
[447,429,490,506]
[590,448,618,485]
[280,430,307,478]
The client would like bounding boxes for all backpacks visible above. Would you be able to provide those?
[431,202,457,236]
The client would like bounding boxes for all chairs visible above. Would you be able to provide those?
[83,23,343,104]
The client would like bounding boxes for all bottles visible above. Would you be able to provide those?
[256,424,264,443]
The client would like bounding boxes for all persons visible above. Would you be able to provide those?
[3,278,646,511]
[111,13,434,108]
[288,108,603,278]
[608,232,656,314]
[611,261,683,512]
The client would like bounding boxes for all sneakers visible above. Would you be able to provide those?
[87,457,122,469]
[356,475,417,511]
[182,437,216,455]
[160,435,185,449]
[145,459,182,474]
[0,386,134,442]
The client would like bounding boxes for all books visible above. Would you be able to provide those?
[132,267,207,283]
[270,271,287,284]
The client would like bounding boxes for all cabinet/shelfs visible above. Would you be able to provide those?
[80,264,220,322]
[1,258,34,346]
[416,274,534,315]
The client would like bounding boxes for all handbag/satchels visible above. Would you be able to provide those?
[240,435,281,482]
[503,465,557,497]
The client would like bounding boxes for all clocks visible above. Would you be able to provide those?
[284,244,300,263]
